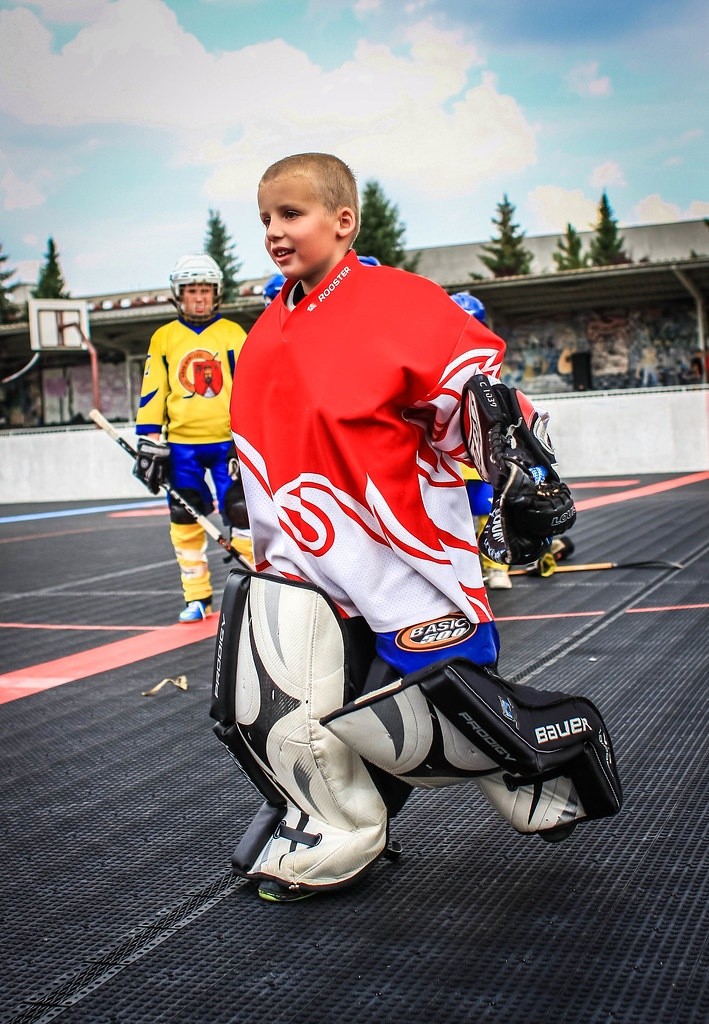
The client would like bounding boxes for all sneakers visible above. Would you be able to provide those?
[258,878,318,902]
[537,821,579,844]
[489,568,512,590]
[180,599,213,622]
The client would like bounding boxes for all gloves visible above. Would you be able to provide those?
[228,440,243,483]
[133,435,171,496]
[477,490,552,564]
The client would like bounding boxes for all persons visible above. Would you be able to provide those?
[133,253,577,619]
[206,152,624,903]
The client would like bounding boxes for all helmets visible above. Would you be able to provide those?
[450,293,486,322]
[171,254,224,297]
[357,256,381,267]
[263,275,286,299]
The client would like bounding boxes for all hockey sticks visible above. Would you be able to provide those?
[481,557,686,582]
[87,409,256,574]
